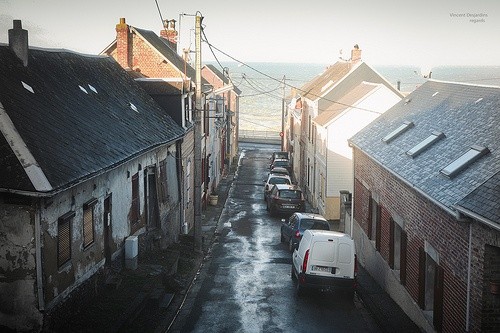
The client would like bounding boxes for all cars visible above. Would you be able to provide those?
[264,150,359,296]
